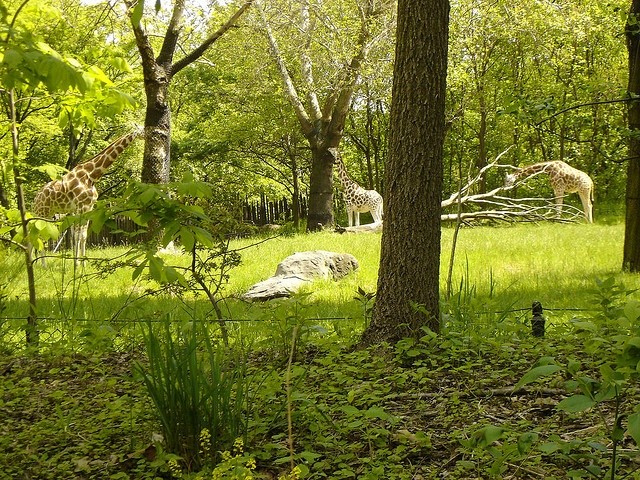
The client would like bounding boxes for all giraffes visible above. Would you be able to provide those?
[503,160,596,225]
[30,121,146,273]
[327,146,384,228]
